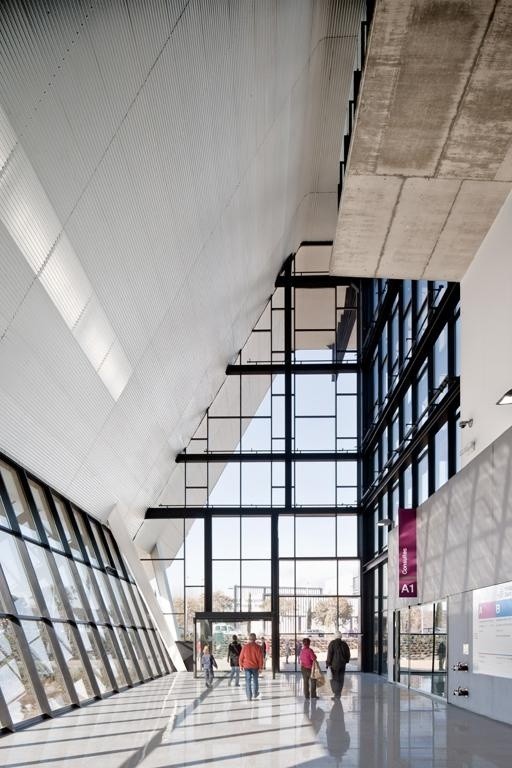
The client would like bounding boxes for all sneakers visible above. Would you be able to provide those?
[305,694,341,700]
[206,681,260,701]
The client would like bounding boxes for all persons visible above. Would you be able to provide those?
[327,699,351,765]
[297,638,320,701]
[436,673,446,696]
[324,631,350,701]
[435,638,447,672]
[199,645,218,689]
[255,635,302,670]
[226,634,243,689]
[302,699,323,759]
[239,632,264,703]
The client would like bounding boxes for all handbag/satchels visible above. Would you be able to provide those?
[327,665,333,680]
